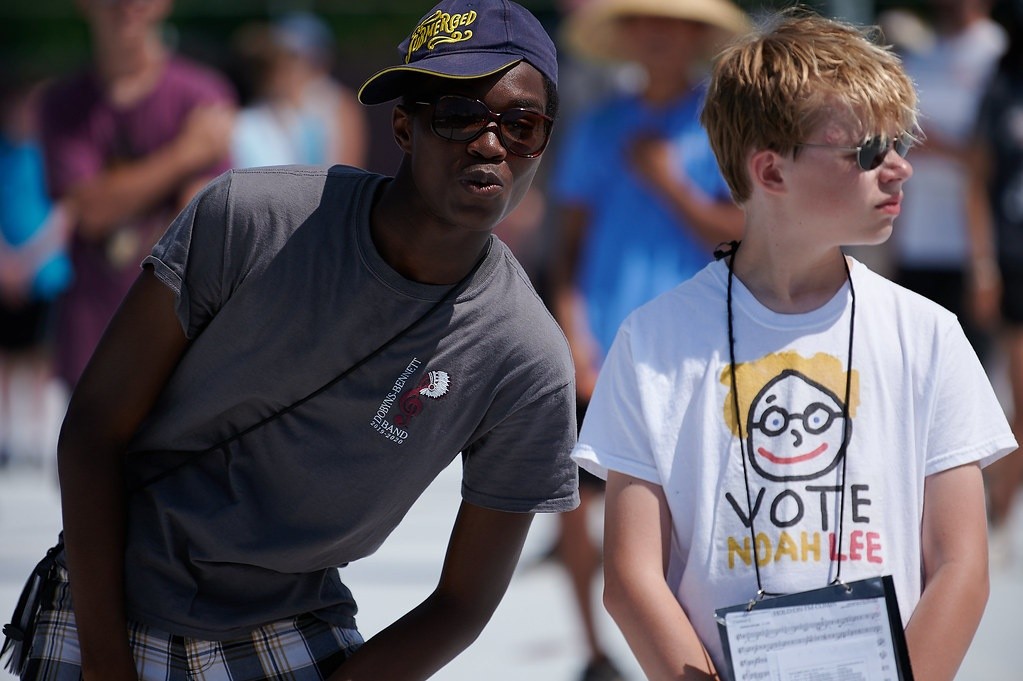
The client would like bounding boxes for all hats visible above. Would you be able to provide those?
[357,0,558,105]
[561,0,760,66]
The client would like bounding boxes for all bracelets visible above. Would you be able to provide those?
[979,271,1003,288]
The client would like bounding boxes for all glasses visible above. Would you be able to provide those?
[766,132,913,173]
[412,94,554,160]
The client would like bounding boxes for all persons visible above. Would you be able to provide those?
[0,0,1023,561]
[18,0,582,681]
[568,17,1020,681]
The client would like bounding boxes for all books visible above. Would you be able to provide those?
[0,558,49,677]
